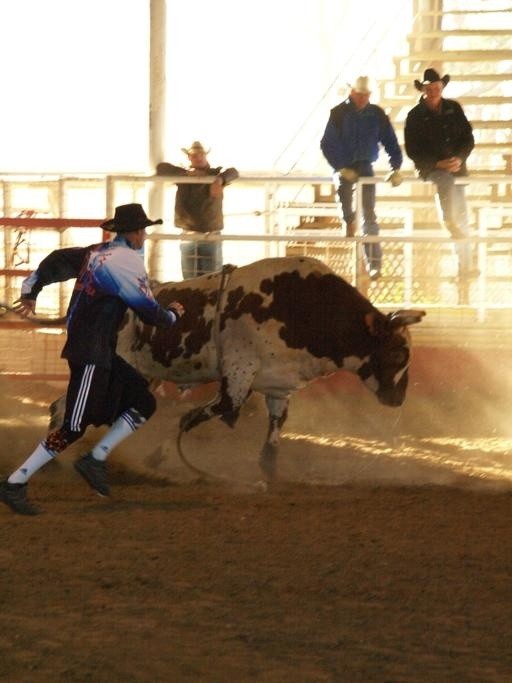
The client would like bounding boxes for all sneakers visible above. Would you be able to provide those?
[75,452,112,496]
[0,481,39,515]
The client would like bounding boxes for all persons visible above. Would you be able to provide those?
[1,203,186,516]
[156,138,242,279]
[404,67,484,281]
[318,72,403,281]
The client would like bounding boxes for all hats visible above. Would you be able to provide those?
[345,75,377,94]
[414,70,451,90]
[181,140,212,154]
[100,203,162,232]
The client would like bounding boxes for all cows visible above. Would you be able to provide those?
[0,251,431,497]
